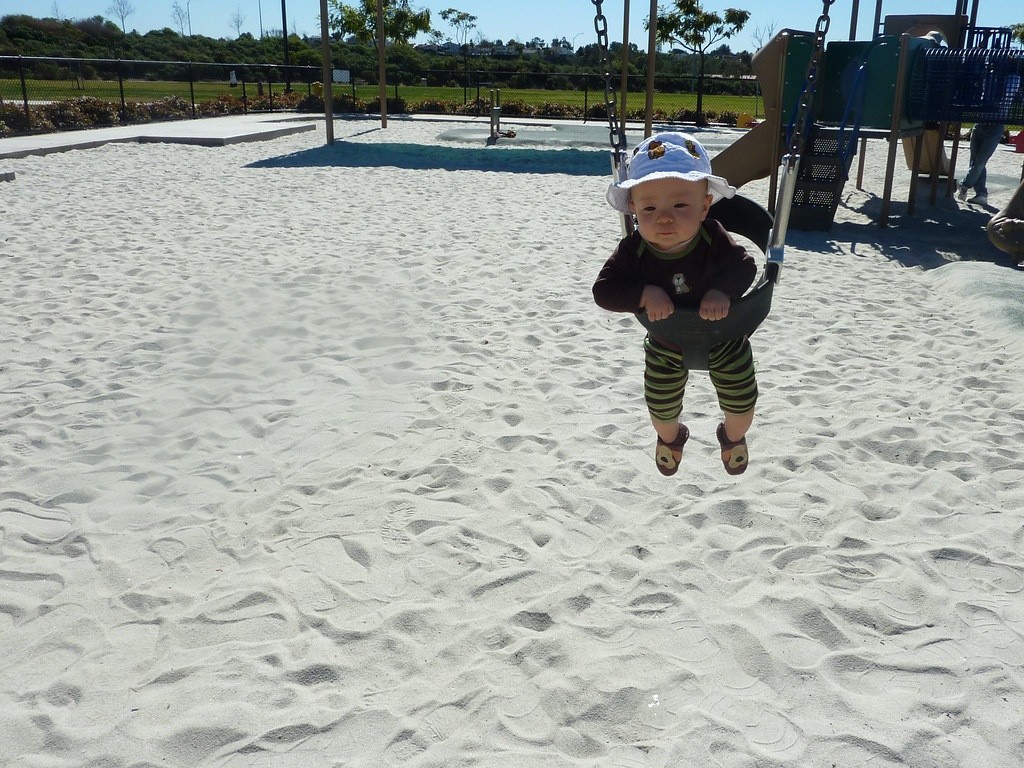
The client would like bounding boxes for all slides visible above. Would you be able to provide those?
[898,118,955,177]
[704,120,783,194]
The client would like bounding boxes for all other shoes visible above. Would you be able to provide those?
[655,422,690,476]
[717,422,748,475]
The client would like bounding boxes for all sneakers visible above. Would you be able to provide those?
[967,195,987,205]
[958,179,968,202]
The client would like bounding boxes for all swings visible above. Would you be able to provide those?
[590,0,835,371]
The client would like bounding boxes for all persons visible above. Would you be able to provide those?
[959,119,1004,204]
[593,131,760,477]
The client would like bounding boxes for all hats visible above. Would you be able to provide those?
[917,31,949,48]
[605,132,736,215]
